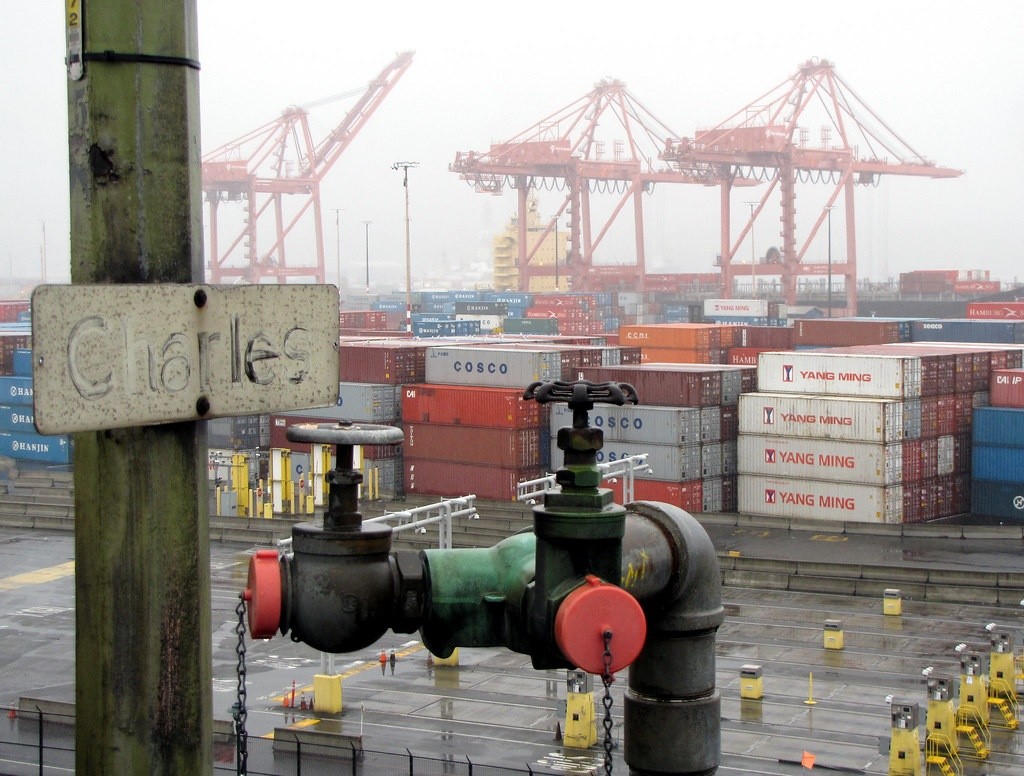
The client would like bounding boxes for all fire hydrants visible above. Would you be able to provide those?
[232,379,725,776]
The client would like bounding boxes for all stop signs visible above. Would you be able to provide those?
[300,480,304,488]
[258,489,262,497]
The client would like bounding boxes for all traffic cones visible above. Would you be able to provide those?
[425,651,433,665]
[427,665,433,680]
[380,661,386,676]
[309,698,314,711]
[390,645,396,661]
[379,647,387,662]
[280,690,290,707]
[389,660,396,676]
[300,692,308,711]
[283,712,289,725]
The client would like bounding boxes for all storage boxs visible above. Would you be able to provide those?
[1,271,1024,530]
